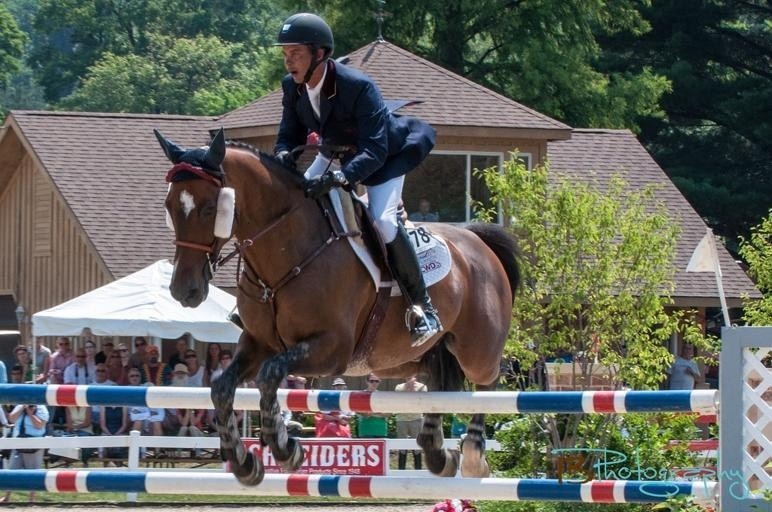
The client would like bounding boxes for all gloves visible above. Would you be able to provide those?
[300,170,348,201]
[273,143,298,171]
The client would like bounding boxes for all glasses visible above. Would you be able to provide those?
[287,374,381,388]
[11,337,233,385]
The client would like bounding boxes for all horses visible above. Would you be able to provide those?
[153,126,520,486]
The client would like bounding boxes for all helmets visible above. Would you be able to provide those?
[271,12,335,59]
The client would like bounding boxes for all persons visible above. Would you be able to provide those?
[0,328,428,503]
[666,342,700,390]
[407,199,438,222]
[273,13,440,340]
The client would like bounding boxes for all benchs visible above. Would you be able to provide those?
[4,422,260,470]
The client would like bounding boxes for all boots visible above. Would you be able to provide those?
[384,219,438,348]
